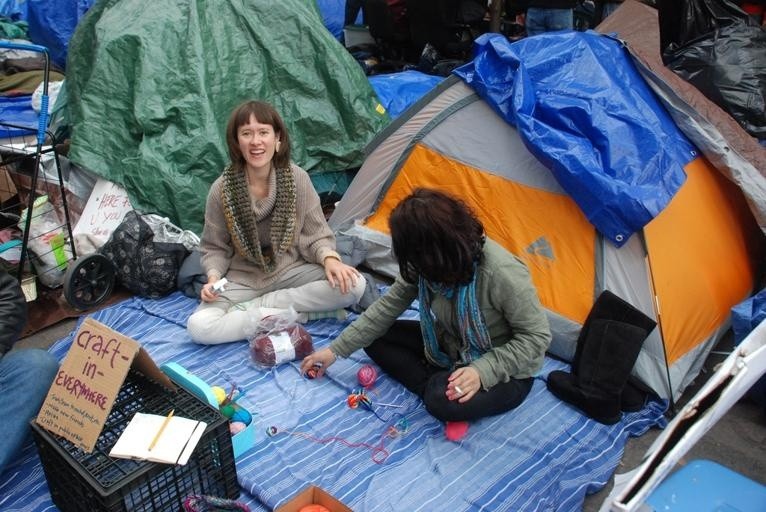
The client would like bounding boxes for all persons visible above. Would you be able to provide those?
[298,186,553,423]
[183,100,368,347]
[338,1,599,76]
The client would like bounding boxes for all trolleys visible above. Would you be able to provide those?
[0,38,116,310]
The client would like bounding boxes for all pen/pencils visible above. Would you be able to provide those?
[147,409,176,452]
[449,381,462,395]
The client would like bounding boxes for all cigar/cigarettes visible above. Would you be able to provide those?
[449,381,464,397]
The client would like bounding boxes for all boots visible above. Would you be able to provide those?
[547,289,658,425]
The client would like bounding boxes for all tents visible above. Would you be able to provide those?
[317,28,766,417]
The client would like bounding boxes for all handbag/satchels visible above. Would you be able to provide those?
[100,210,201,300]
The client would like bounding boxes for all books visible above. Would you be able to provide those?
[108,411,209,466]
